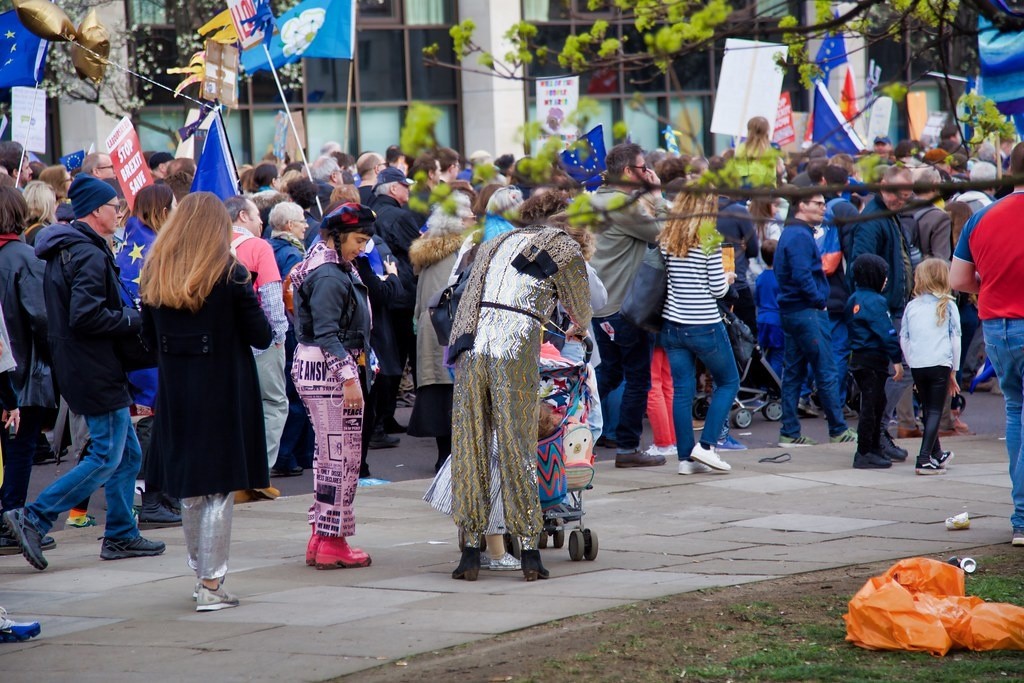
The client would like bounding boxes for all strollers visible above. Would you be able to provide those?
[458,329,600,561]
[693,298,782,428]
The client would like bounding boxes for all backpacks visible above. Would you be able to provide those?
[563,416,594,491]
[900,207,942,268]
[815,197,851,274]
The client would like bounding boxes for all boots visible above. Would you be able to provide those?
[306,523,372,569]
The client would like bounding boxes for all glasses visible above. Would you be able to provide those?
[809,201,828,209]
[381,162,390,167]
[295,219,307,227]
[629,165,648,173]
[455,165,462,169]
[105,203,121,214]
[98,164,114,170]
[64,175,74,182]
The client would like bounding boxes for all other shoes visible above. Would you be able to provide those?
[597,397,969,474]
[1,487,182,569]
[234,417,409,502]
[453,547,550,582]
[1011,528,1024,546]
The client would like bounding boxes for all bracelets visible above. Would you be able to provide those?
[343,380,355,386]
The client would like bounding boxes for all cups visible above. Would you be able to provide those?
[722,243,736,273]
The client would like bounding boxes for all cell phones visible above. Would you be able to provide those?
[386,255,390,265]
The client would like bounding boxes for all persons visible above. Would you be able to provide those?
[0,115,1023,642]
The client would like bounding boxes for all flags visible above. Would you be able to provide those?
[0,0,866,412]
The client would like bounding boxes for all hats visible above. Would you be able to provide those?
[874,134,892,145]
[378,165,418,186]
[470,150,491,159]
[68,172,117,216]
[320,202,378,227]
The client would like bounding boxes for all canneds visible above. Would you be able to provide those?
[948,556,977,573]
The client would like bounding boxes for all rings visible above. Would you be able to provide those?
[354,403,358,406]
[349,404,353,407]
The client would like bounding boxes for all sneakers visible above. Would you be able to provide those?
[0,607,40,643]
[194,575,239,612]
[395,388,417,407]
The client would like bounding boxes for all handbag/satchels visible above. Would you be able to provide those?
[727,319,753,361]
[618,247,668,334]
[428,265,475,346]
